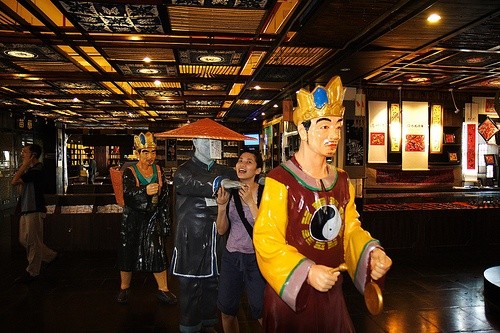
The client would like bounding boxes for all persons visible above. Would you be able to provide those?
[152,119,257,333]
[11,144,61,281]
[117,133,177,305]
[253,77,391,333]
[217,150,264,333]
[87,153,96,185]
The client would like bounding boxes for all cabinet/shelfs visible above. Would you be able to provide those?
[262,116,286,168]
[366,123,462,172]
